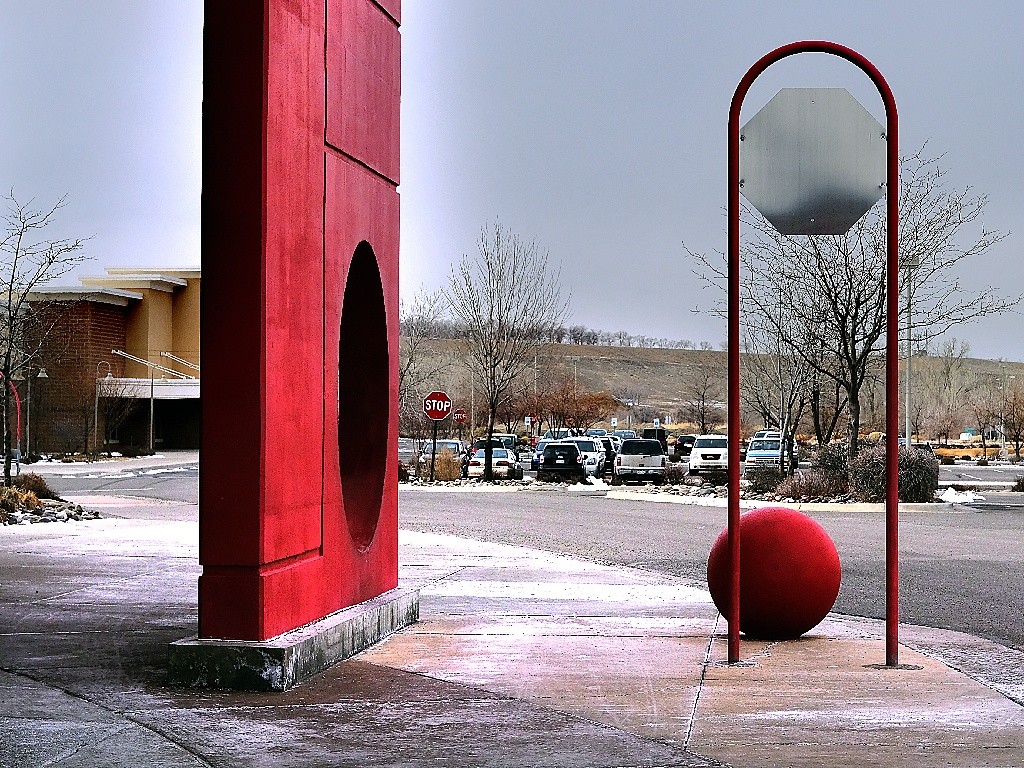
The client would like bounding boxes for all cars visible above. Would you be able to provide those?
[419,439,467,470]
[467,447,517,480]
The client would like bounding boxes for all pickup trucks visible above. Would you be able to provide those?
[612,438,666,486]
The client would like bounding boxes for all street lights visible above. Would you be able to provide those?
[26,355,49,459]
[467,329,478,437]
[532,344,540,403]
[94,361,116,460]
[150,364,168,449]
[898,255,919,445]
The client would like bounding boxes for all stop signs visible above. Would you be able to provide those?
[452,409,467,423]
[423,390,452,421]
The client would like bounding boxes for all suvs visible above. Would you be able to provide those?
[674,434,700,455]
[739,428,801,474]
[468,433,518,459]
[897,437,936,460]
[688,434,729,475]
[537,441,588,483]
[531,427,669,471]
